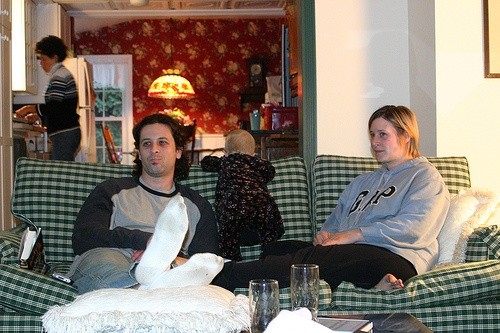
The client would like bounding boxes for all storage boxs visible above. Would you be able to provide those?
[273,106,299,131]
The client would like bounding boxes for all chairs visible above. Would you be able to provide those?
[100,123,122,164]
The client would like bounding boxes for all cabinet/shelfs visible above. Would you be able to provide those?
[283,0,302,105]
[0,2,72,229]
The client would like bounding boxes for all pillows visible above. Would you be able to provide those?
[433,189,500,264]
[37,285,250,332]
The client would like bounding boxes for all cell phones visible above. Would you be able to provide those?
[52,273,71,284]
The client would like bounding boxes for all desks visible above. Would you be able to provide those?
[246,130,301,157]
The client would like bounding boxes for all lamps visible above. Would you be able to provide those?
[147,18,196,100]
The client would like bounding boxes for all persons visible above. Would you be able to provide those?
[68,115,217,293]
[261,105,449,292]
[200,129,286,263]
[16,35,82,162]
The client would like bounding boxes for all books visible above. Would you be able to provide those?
[317,318,372,333]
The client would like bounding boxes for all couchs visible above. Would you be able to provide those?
[1,157,500,333]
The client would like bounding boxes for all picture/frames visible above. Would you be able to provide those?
[483,0,500,78]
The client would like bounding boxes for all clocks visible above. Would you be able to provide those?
[237,56,268,108]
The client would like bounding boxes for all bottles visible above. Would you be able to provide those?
[259,103,272,130]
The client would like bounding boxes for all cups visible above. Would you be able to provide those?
[249,279,280,333]
[290,264,319,322]
[249,112,260,130]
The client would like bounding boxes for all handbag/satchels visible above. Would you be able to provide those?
[18,227,44,270]
